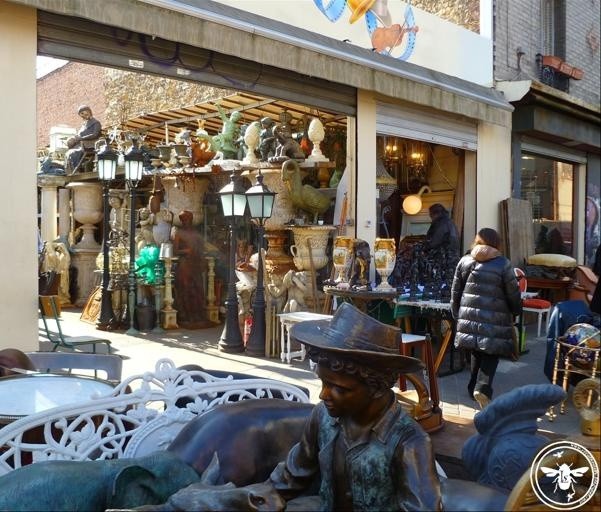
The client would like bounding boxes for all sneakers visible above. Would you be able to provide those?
[468,382,493,410]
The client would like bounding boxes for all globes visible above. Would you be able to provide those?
[549,323,601,422]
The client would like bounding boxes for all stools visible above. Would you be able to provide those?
[278,311,426,391]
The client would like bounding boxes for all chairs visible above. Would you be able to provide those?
[514,268,552,338]
[38,295,111,379]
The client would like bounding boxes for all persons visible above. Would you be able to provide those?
[174,211,207,322]
[423,203,459,257]
[108,193,128,233]
[66,105,101,177]
[135,207,155,246]
[258,117,277,162]
[264,301,443,511]
[213,98,242,153]
[451,228,521,409]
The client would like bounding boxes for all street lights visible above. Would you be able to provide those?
[122,144,146,333]
[243,167,279,354]
[95,146,119,331]
[216,167,246,354]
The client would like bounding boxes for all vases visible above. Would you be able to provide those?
[289,223,398,294]
[156,144,188,162]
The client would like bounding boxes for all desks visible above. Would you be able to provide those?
[527,277,571,303]
